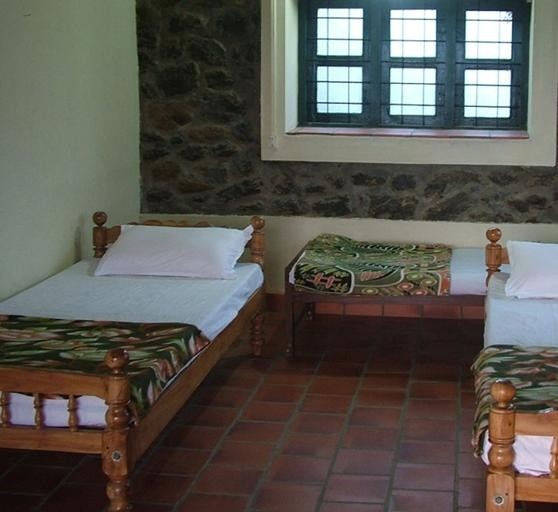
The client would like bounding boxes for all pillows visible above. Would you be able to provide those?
[93,224,253,280]
[503,240,558,301]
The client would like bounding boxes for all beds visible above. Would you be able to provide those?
[284,241,558,359]
[1,210,267,511]
[486,228,558,512]
